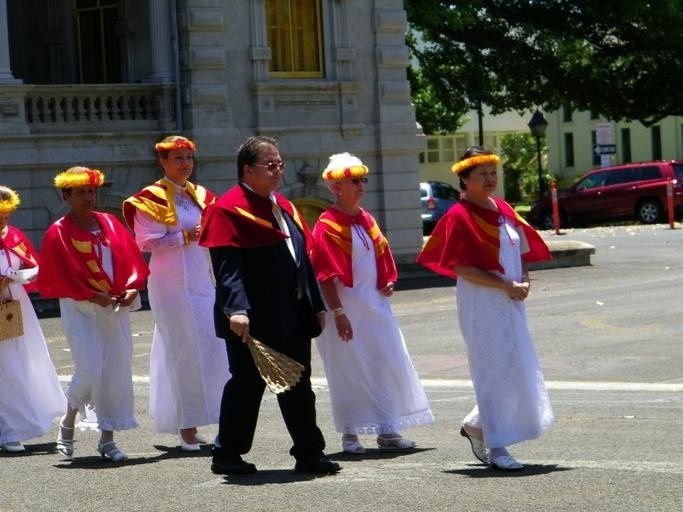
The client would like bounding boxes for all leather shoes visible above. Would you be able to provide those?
[211,451,257,474]
[295,456,339,474]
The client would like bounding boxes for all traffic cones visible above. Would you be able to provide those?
[550,181,560,235]
[666,176,675,229]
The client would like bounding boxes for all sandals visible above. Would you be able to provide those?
[376,436,415,450]
[342,437,365,453]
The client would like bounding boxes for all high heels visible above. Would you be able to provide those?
[57,416,75,457]
[178,429,201,450]
[4,441,27,452]
[194,432,208,443]
[97,442,128,462]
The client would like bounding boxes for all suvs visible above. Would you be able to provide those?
[531,160,682,229]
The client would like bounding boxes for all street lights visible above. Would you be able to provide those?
[528,109,549,230]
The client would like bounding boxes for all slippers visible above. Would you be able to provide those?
[460,426,491,462]
[489,451,524,470]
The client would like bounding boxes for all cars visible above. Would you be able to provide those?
[418,180,461,236]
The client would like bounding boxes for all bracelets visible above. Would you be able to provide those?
[182,228,191,247]
[328,308,346,322]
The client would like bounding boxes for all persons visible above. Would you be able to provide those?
[196,135,343,478]
[122,137,233,451]
[314,153,436,454]
[36,165,151,462]
[0,187,69,456]
[417,146,556,472]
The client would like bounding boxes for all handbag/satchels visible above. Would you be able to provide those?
[0,276,24,341]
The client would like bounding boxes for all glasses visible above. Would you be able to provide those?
[336,177,369,184]
[248,162,284,171]
